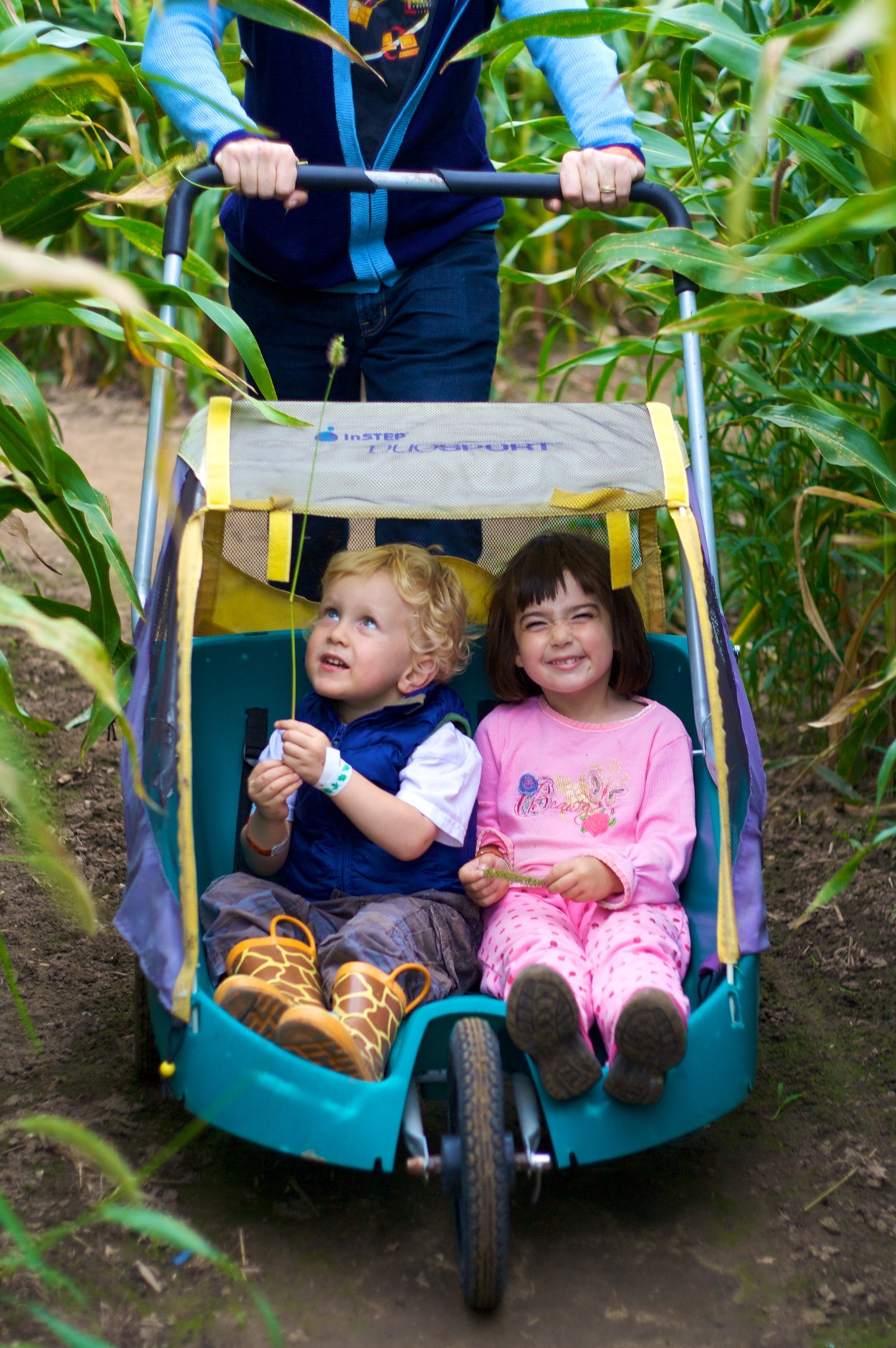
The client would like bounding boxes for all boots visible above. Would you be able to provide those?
[211,914,326,1043]
[276,963,431,1081]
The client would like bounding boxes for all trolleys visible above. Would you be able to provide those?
[135,165,764,1315]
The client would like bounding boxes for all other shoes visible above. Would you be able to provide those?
[600,987,687,1113]
[506,963,605,1104]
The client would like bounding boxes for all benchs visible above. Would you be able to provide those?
[153,629,713,1081]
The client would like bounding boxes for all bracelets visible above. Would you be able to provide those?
[246,815,291,855]
[475,852,511,886]
[313,747,352,795]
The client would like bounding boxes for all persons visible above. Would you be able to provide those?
[140,0,644,601]
[458,529,696,1106]
[197,544,481,1081]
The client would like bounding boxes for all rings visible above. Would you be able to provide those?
[599,185,614,193]
